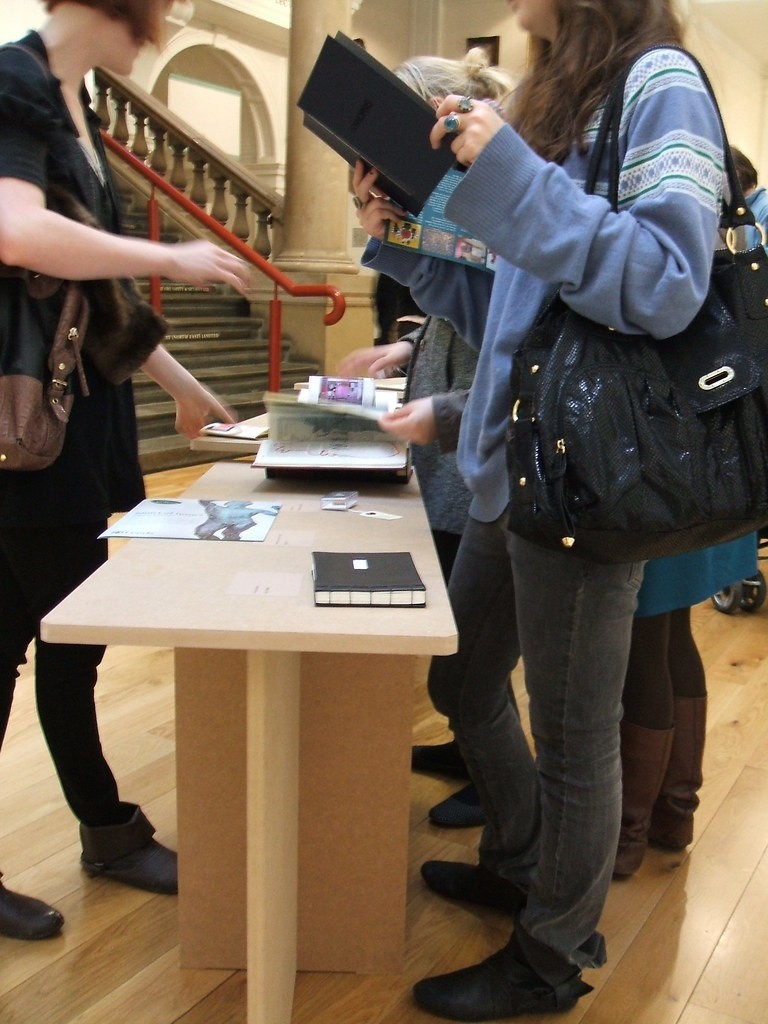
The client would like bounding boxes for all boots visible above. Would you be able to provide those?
[0,889,69,943]
[74,810,185,899]
[660,695,711,857]
[616,719,667,877]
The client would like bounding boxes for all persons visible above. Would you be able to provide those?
[336,0,768,1021]
[0,0,257,939]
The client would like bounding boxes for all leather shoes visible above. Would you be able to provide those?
[419,857,527,910]
[412,946,592,1009]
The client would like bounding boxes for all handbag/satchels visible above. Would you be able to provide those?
[0,260,88,478]
[504,41,768,562]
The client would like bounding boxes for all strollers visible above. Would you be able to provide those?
[710,526,768,614]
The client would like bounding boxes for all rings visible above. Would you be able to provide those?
[352,194,371,209]
[458,95,473,113]
[445,111,461,133]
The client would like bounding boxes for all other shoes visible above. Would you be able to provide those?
[410,744,485,773]
[431,791,494,822]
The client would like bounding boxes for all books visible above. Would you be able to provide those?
[294,31,460,218]
[311,551,426,607]
[190,374,414,483]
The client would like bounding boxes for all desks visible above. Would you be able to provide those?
[40,456,460,1024]
